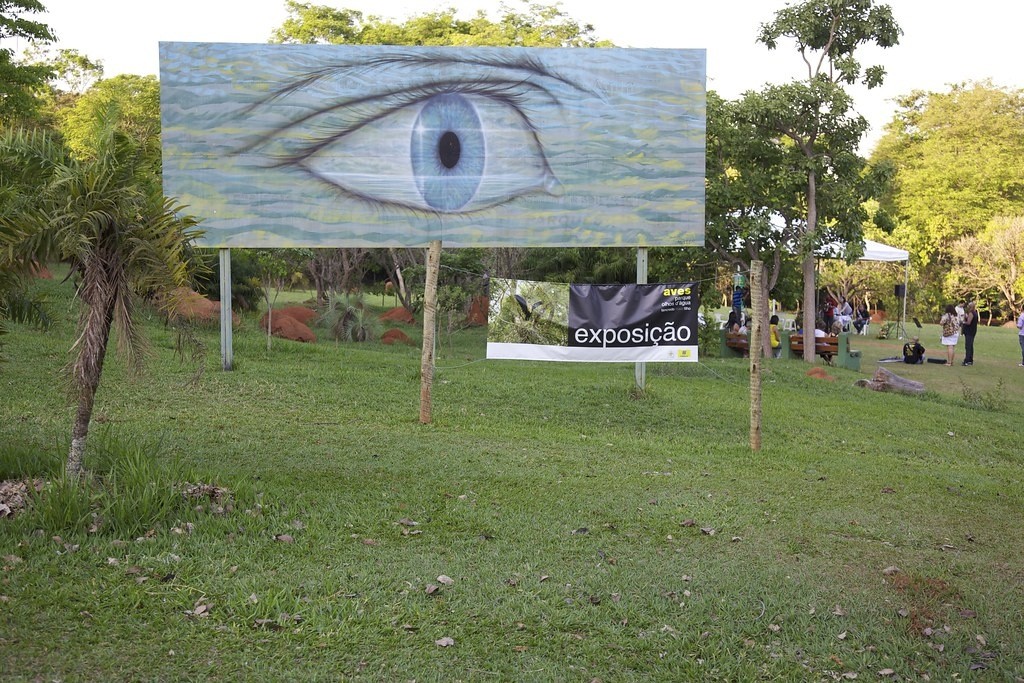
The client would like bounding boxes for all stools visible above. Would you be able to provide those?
[783,318,796,331]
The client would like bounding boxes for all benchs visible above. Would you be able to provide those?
[779,330,862,374]
[719,328,778,357]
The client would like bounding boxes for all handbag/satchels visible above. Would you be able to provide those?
[943,313,954,337]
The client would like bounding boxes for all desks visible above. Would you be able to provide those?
[834,315,850,333]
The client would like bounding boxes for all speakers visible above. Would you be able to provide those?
[895,285,905,296]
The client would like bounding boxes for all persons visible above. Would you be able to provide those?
[737,318,752,358]
[795,316,804,359]
[954,300,966,336]
[960,302,977,366]
[820,294,837,335]
[815,320,832,366]
[838,296,852,331]
[903,336,925,365]
[769,315,782,358]
[853,304,870,336]
[724,312,739,334]
[732,286,745,328]
[940,305,963,366]
[1016,304,1024,366]
[828,321,843,337]
[974,310,977,313]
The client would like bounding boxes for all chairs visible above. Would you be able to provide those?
[854,316,872,334]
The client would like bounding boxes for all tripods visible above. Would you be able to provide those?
[887,296,910,340]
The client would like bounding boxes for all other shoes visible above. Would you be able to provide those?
[854,332,859,335]
[828,362,831,365]
[943,362,952,367]
[829,358,832,361]
[961,362,972,366]
[1019,364,1024,367]
[859,330,864,335]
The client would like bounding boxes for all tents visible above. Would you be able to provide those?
[705,206,909,340]
